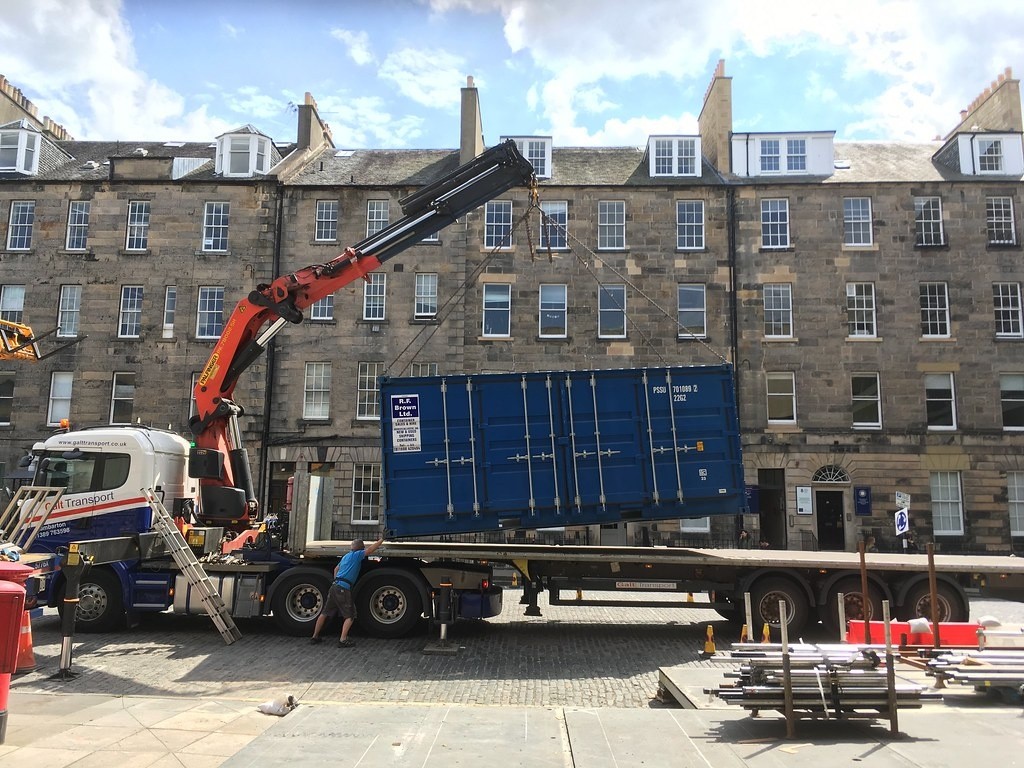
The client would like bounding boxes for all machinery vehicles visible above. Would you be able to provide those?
[0,139,1023,657]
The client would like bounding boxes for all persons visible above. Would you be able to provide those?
[735,529,777,550]
[852,532,921,555]
[310,538,383,647]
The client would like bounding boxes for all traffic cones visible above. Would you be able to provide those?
[508,571,520,589]
[574,590,583,601]
[687,592,694,603]
[760,622,771,644]
[11,610,46,675]
[739,623,748,643]
[697,625,720,658]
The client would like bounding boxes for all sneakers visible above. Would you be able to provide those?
[338,639,355,648]
[311,638,322,643]
